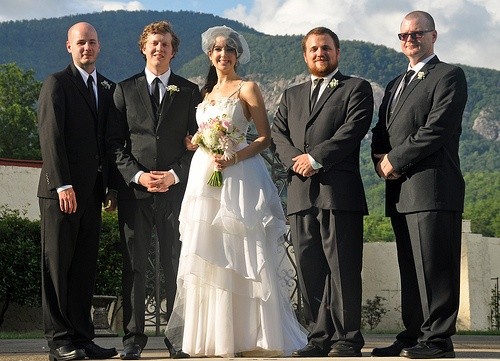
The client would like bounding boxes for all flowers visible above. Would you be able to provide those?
[101,81,111,94]
[165,84,181,96]
[328,79,340,87]
[417,72,425,80]
[194,116,244,187]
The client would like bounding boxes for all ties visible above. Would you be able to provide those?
[392,70,416,110]
[151,78,160,109]
[309,78,324,112]
[87,75,96,111]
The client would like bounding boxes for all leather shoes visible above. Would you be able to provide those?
[121,350,142,360]
[171,351,192,358]
[372,331,418,356]
[327,349,362,357]
[49,346,83,361]
[83,343,119,360]
[292,344,327,356]
[402,337,456,357]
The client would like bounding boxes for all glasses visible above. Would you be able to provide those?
[398,30,435,40]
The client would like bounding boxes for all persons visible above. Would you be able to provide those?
[36,22,121,361]
[370,10,468,358]
[104,20,202,360]
[164,25,308,358]
[269,27,375,357]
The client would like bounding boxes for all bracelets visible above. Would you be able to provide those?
[234,152,237,164]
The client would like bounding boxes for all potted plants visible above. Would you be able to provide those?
[92,211,122,335]
